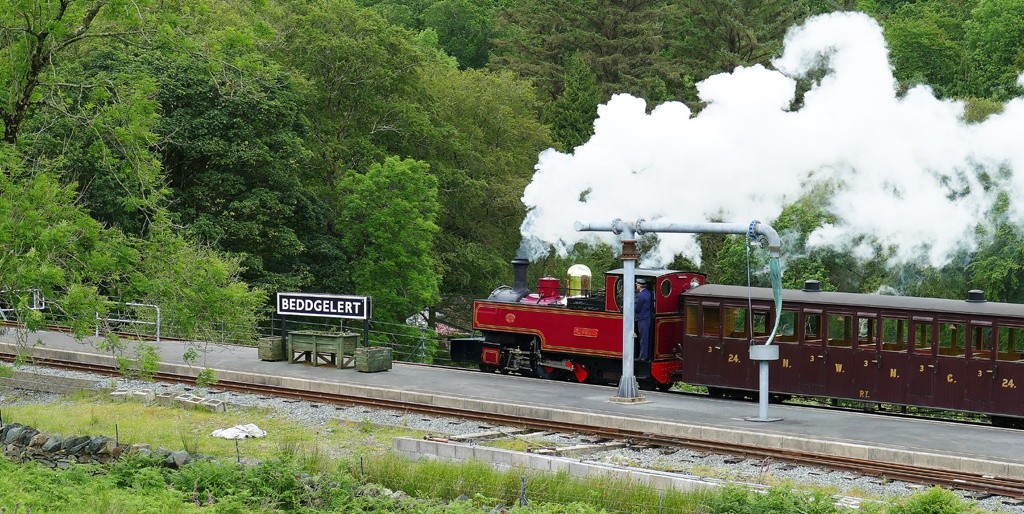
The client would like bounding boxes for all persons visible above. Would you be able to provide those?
[634,279,651,361]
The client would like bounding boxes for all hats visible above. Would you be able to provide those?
[635,279,646,286]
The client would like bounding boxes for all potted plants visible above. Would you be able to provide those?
[287,324,361,369]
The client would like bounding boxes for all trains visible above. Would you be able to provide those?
[449,253,1024,429]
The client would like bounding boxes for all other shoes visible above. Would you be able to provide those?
[634,357,645,361]
